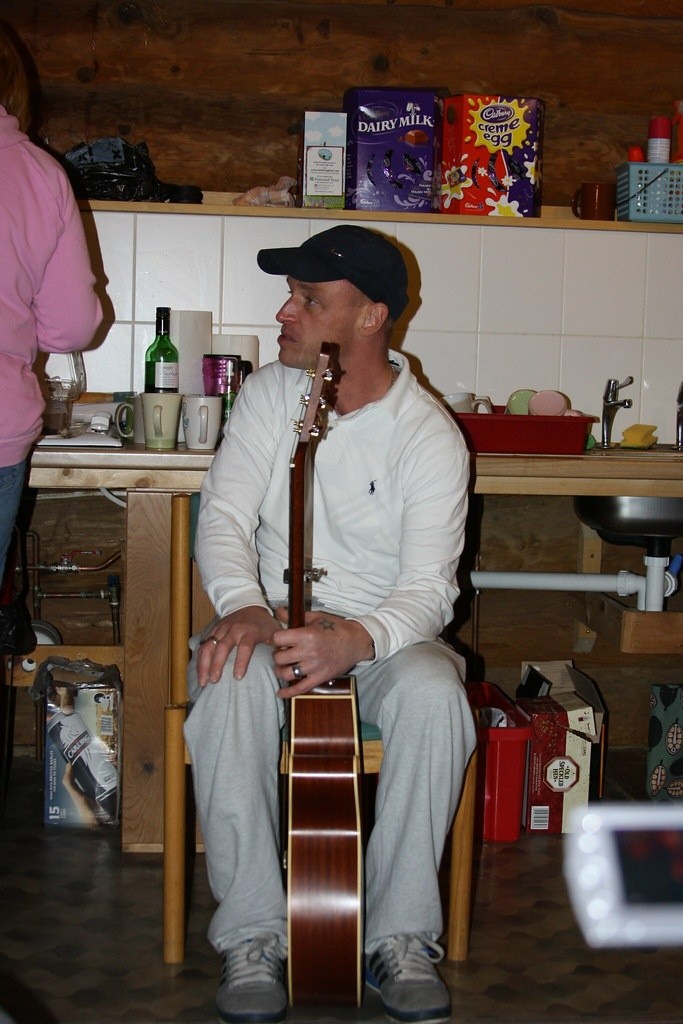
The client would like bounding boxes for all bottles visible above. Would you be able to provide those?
[628,114,672,165]
[144,306,180,394]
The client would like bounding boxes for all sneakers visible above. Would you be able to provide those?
[213,935,289,1024]
[362,932,453,1024]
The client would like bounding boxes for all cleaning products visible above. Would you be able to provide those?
[648,113,670,215]
[670,99,683,163]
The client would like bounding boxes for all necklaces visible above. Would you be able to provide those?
[388,365,395,390]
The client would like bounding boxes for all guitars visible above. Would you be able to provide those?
[277,338,366,1019]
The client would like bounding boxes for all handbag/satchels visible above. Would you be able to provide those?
[63,137,203,203]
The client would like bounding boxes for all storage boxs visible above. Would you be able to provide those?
[296,87,545,217]
[451,406,600,454]
[463,660,605,843]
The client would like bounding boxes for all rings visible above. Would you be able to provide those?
[293,663,303,679]
[207,636,218,647]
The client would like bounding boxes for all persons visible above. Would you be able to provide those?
[1,28,106,570]
[184,227,481,1021]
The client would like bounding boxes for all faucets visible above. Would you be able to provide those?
[597,375,634,450]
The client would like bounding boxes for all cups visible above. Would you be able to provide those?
[570,182,617,222]
[115,395,146,444]
[181,395,222,451]
[139,392,184,449]
[436,388,494,414]
[503,389,584,417]
[203,353,254,451]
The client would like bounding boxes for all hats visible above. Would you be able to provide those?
[257,223,409,321]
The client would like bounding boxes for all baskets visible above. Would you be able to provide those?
[615,160,683,223]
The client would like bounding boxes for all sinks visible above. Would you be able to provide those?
[576,447,683,540]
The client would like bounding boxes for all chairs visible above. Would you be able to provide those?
[163,492,479,963]
